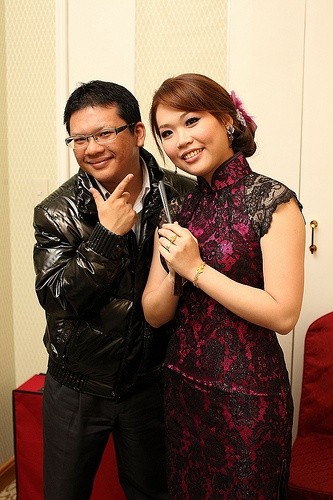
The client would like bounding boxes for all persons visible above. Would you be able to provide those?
[31,80,198,500]
[140,73,305,500]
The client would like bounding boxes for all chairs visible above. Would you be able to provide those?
[277,312,333,500]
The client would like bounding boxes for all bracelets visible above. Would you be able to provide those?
[193,262,206,288]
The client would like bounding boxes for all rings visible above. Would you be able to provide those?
[171,234,177,243]
[166,243,172,250]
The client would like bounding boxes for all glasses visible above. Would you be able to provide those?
[65,122,135,149]
